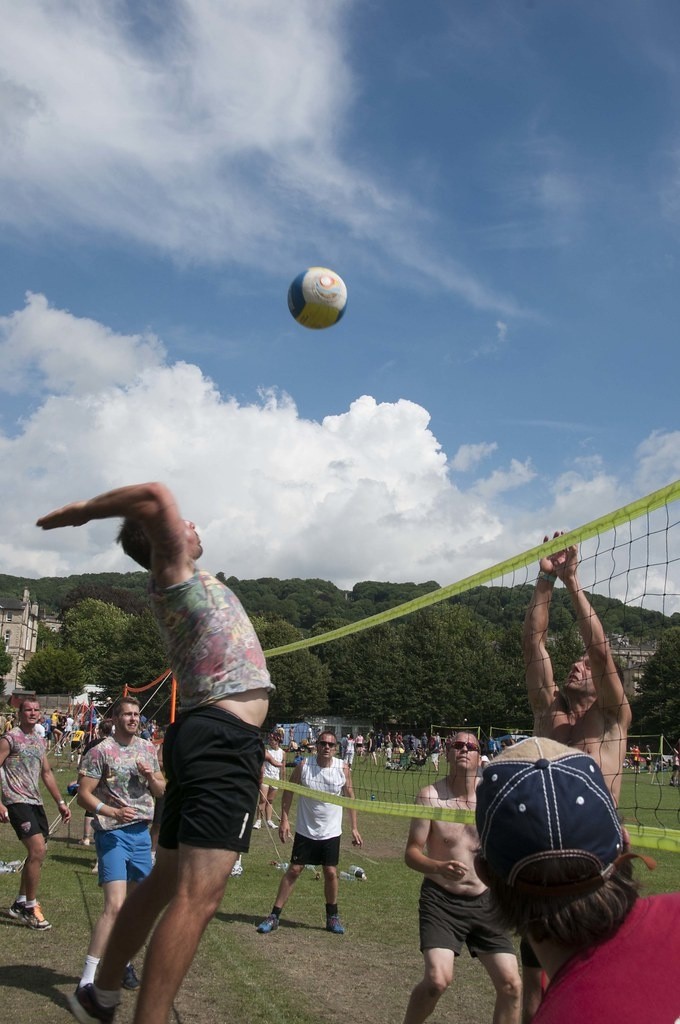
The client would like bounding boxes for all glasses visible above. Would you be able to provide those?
[448,742,480,753]
[316,741,336,748]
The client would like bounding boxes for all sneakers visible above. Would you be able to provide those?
[258,914,278,934]
[10,901,24,919]
[123,964,141,990]
[326,913,344,934]
[19,904,52,932]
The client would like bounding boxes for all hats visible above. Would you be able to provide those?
[474,736,656,897]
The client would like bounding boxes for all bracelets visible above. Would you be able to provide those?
[538,571,556,583]
[57,800,65,804]
[95,802,105,814]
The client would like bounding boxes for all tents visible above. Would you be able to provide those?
[270,722,317,746]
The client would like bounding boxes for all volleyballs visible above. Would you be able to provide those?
[286,266,348,331]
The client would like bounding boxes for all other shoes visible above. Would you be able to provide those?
[252,820,261,830]
[79,838,90,846]
[67,982,116,1024]
[229,865,241,877]
[267,821,279,830]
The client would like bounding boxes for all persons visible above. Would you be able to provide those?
[77,720,112,846]
[623,744,680,787]
[0,709,164,766]
[0,697,72,931]
[519,532,632,1024]
[272,723,530,772]
[257,730,363,934]
[472,735,680,1024]
[404,732,523,1024]
[75,696,166,997]
[252,728,286,830]
[36,481,275,1024]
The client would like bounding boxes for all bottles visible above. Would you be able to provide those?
[338,871,355,882]
[348,865,367,881]
[276,863,289,869]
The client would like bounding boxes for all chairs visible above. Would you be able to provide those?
[408,755,427,769]
